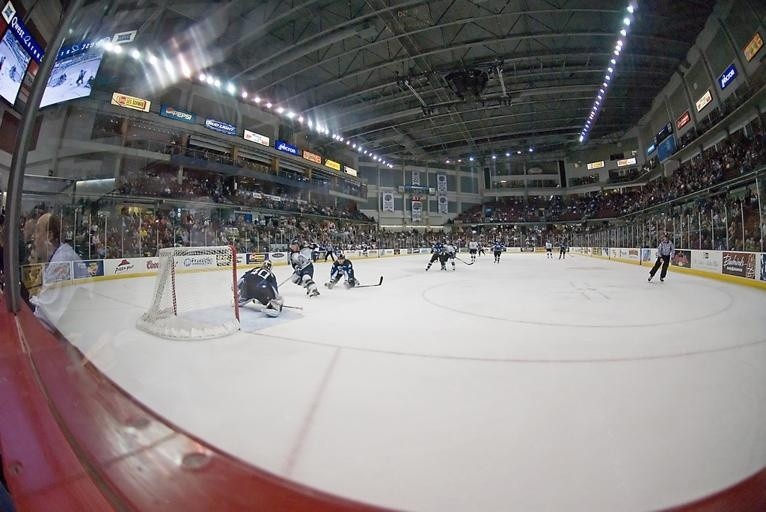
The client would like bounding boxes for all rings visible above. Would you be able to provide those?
[8,64,16,82]
[51,72,67,88]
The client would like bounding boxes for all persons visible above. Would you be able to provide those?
[647,234,675,282]
[83,75,95,89]
[0,56,6,71]
[544,239,553,258]
[442,162,672,256]
[230,259,283,317]
[490,241,505,264]
[424,239,444,271]
[73,68,87,86]
[672,83,765,253]
[467,237,478,263]
[30,212,93,347]
[375,225,442,255]
[558,240,565,260]
[324,254,360,290]
[0,144,375,269]
[284,235,321,297]
[441,236,454,270]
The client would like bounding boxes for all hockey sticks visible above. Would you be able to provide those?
[455,257,474,265]
[353,276,384,287]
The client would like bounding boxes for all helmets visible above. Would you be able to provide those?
[262,259,273,271]
[288,239,301,247]
[337,253,346,264]
